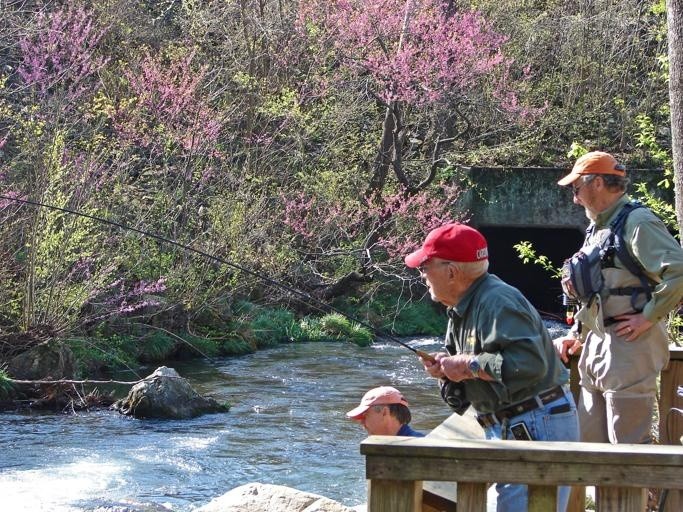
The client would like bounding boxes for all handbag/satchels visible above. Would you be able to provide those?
[559,245,607,302]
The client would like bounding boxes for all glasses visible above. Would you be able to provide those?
[571,181,586,196]
[416,261,450,273]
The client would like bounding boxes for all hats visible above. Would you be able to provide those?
[404,222,489,267]
[347,386,409,421]
[557,151,626,187]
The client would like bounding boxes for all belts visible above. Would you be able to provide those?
[476,385,565,429]
[604,311,640,328]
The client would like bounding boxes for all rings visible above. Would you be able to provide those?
[627,327,632,332]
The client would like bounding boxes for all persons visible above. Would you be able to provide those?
[346,385,426,438]
[404,222,581,512]
[552,151,683,445]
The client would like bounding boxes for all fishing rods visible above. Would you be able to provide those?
[1,195,470,415]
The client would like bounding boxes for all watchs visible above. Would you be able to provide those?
[468,355,480,378]
